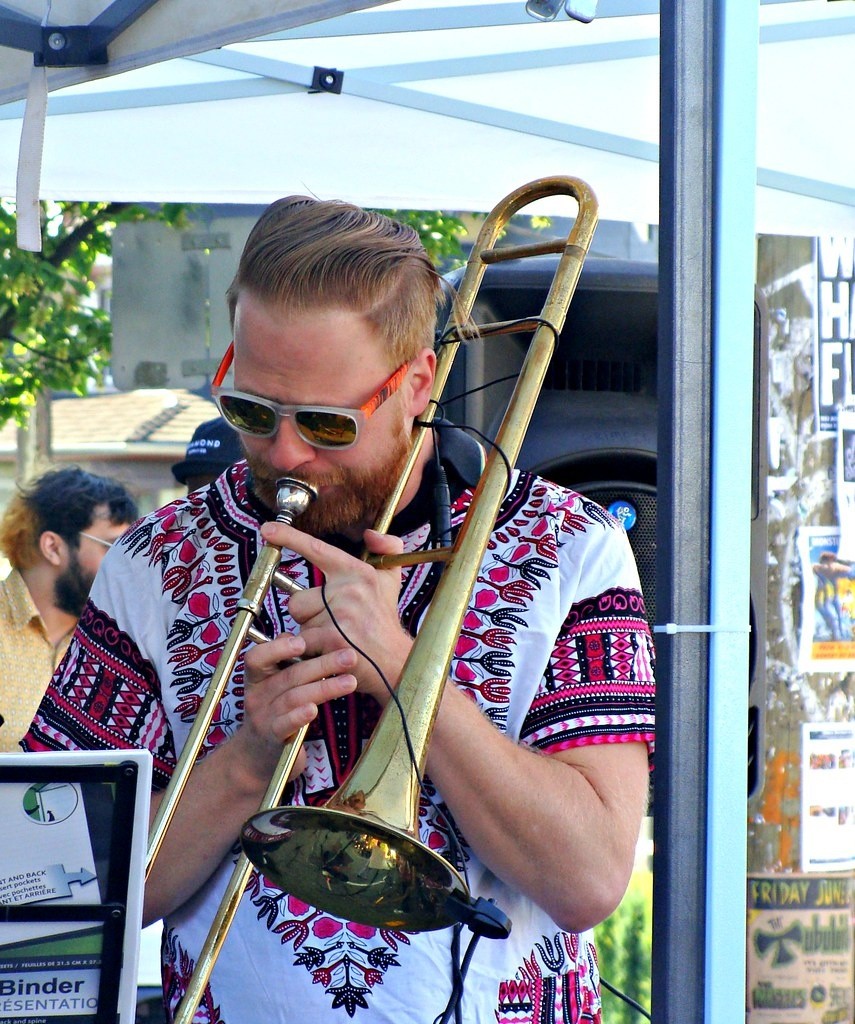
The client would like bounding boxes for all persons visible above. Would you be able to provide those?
[18,196,660,1024]
[0,465,141,749]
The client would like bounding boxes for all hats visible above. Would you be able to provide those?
[171,418,246,485]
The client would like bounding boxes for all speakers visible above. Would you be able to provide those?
[32,26,107,66]
[427,252,769,805]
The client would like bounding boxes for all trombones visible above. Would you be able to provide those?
[139,175,599,1023]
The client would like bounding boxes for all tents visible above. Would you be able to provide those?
[1,0,855,1024]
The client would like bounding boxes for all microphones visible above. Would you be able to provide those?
[431,467,451,546]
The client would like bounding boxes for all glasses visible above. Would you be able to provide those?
[211,340,426,451]
